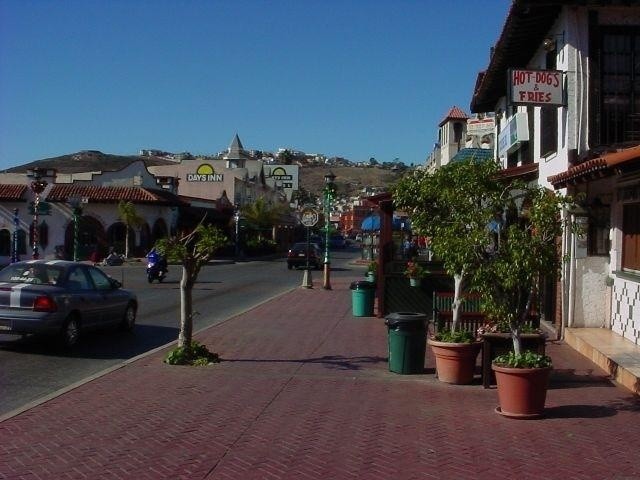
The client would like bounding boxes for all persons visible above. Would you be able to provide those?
[404,236,415,255]
[145,245,168,278]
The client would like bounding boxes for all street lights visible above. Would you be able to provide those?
[232,200,243,262]
[11,181,48,264]
[323,169,338,287]
[72,206,83,262]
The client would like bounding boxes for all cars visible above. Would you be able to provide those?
[0,259,138,347]
[286,228,363,270]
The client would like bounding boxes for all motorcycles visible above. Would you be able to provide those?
[145,252,168,283]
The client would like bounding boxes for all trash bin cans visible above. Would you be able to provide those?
[349,281,377,317]
[385,311,428,374]
